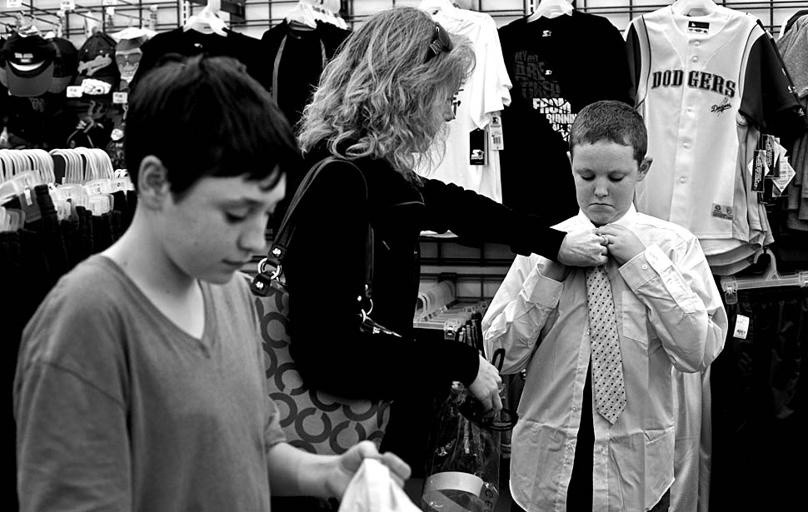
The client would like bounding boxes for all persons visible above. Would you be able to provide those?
[480,100,730,512]
[270,5,610,511]
[12,52,415,510]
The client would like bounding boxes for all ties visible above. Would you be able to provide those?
[581,265,627,426]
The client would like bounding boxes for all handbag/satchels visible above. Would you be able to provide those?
[240,155,403,457]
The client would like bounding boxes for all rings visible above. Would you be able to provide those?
[602,235,609,245]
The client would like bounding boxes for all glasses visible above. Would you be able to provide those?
[423,22,453,64]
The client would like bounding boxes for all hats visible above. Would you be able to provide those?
[1,33,148,100]
